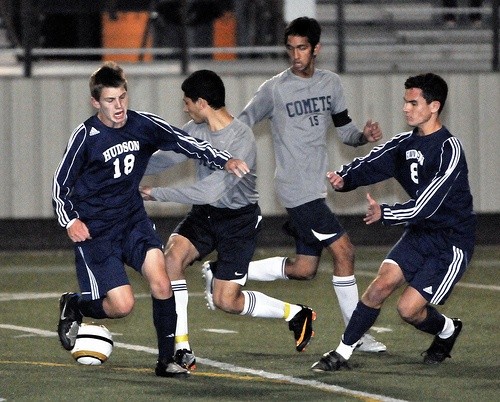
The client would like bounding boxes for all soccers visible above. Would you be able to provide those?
[70,322,114,365]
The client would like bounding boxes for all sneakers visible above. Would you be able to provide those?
[201,260,216,311]
[57,291,81,350]
[288,303,315,351]
[311,351,351,374]
[423,319,462,365]
[174,349,196,371]
[154,356,187,375]
[343,333,386,352]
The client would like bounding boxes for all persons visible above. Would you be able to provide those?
[313,74,474,371]
[137,69,317,369]
[202,16,387,353]
[52,63,249,377]
